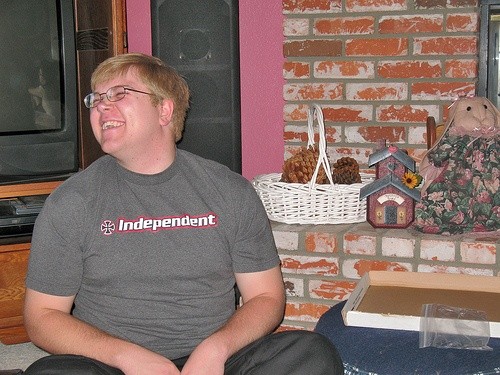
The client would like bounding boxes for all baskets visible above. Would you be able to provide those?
[251,103,376,226]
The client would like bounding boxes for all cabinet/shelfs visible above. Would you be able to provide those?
[0,181,65,345]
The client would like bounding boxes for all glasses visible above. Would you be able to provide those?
[83,85,155,108]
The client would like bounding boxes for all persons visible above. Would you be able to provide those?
[24,53,345,375]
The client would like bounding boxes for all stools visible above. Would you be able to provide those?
[314,299,500,375]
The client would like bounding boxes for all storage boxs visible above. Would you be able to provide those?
[340,270,500,338]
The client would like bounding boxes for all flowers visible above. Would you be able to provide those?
[401,172,417,189]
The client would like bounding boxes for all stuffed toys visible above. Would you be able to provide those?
[417,98,500,236]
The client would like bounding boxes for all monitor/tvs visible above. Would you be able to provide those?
[0,0,79,185]
